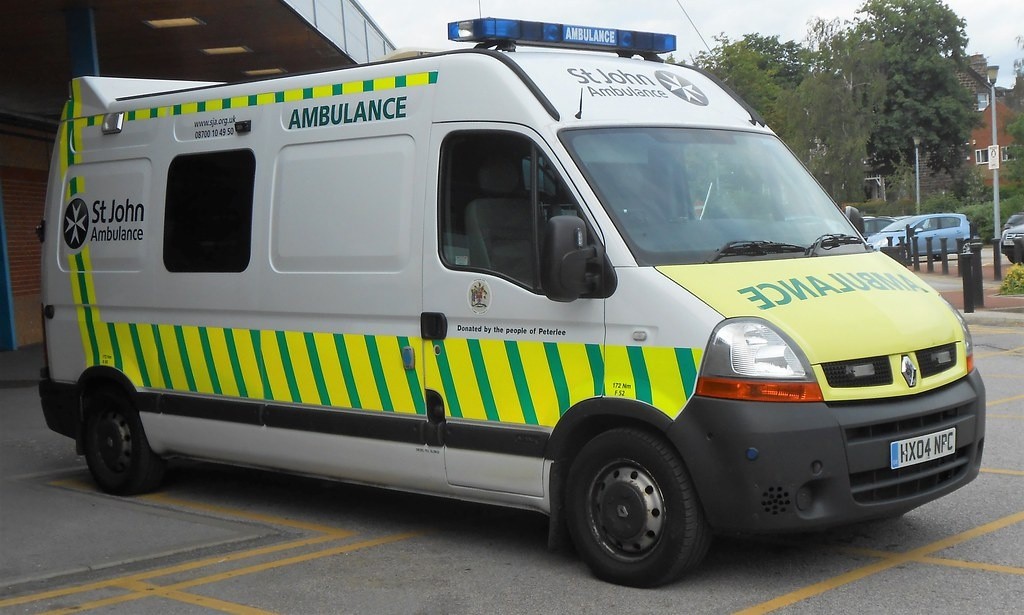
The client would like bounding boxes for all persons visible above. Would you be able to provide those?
[841,202,865,234]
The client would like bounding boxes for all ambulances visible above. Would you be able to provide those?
[33,15,987,592]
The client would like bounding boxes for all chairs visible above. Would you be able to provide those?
[465,199,546,290]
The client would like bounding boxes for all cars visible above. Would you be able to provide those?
[866,211,979,261]
[1000,213,1024,264]
[862,214,911,243]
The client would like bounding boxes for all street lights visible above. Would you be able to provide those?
[986,65,1001,259]
[911,133,921,216]
